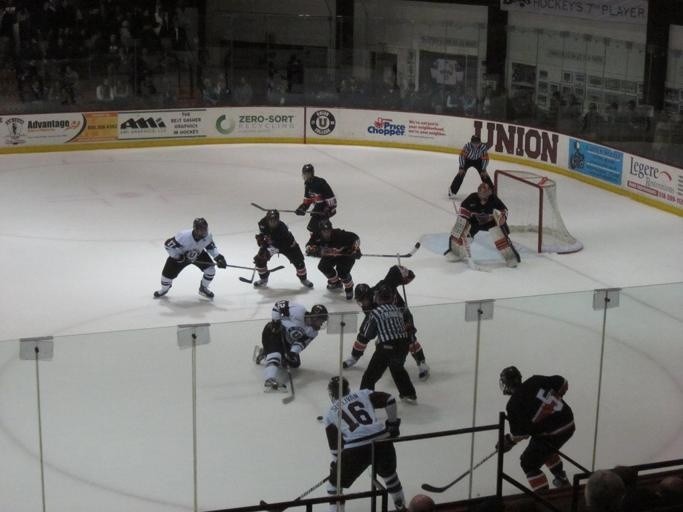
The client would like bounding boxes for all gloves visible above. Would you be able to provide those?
[495,433,516,453]
[385,418,400,436]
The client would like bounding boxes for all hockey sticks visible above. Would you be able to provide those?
[185,260,283,272]
[321,243,419,257]
[277,323,294,403]
[251,202,325,214]
[260,477,328,512]
[447,187,490,271]
[421,440,514,493]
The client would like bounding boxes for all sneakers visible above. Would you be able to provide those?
[255,347,264,365]
[253,278,268,287]
[199,286,214,297]
[398,387,416,399]
[264,378,286,390]
[416,361,428,379]
[342,355,356,369]
[301,280,353,300]
[154,289,168,297]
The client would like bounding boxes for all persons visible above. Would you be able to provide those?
[304,218,363,293]
[254,209,314,288]
[494,365,577,492]
[323,376,406,512]
[295,164,338,245]
[341,265,431,378]
[450,182,519,263]
[360,288,418,399]
[153,216,227,298]
[0,0,683,170]
[408,469,683,512]
[450,135,495,194]
[255,299,330,386]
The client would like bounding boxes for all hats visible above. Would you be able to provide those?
[471,134,480,143]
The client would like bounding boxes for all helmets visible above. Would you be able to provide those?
[303,164,314,177]
[193,217,208,229]
[319,220,332,232]
[478,183,492,201]
[267,209,279,221]
[310,305,328,321]
[327,375,349,404]
[355,283,395,307]
[498,366,522,394]
